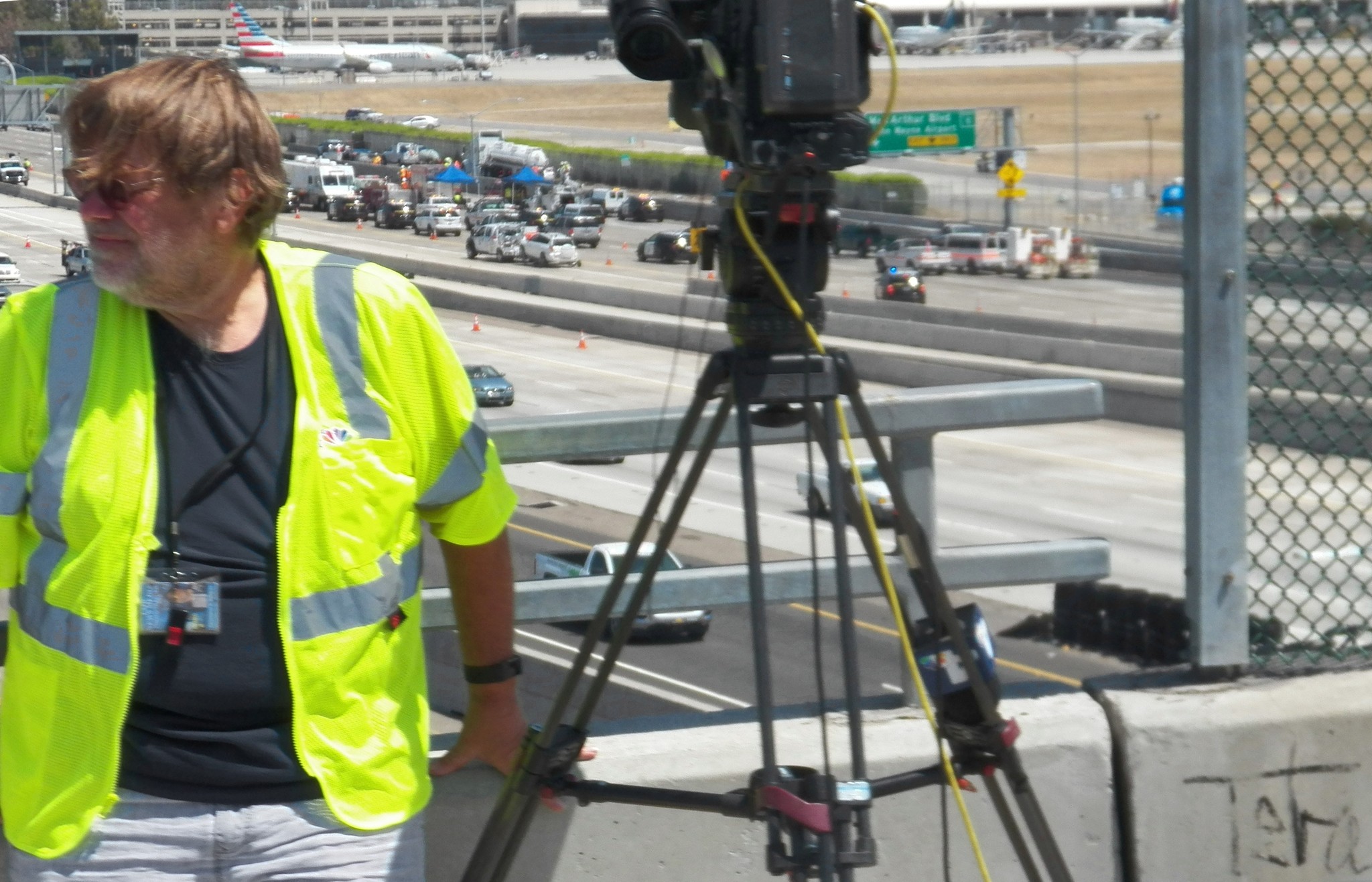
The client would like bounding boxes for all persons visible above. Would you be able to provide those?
[372,152,381,165]
[504,182,527,198]
[453,191,463,205]
[0,58,599,882]
[398,164,411,190]
[22,157,31,170]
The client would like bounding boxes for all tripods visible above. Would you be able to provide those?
[458,353,1079,881]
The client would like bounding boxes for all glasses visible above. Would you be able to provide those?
[62,163,172,210]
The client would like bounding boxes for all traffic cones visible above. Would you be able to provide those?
[575,329,591,352]
[840,284,850,297]
[706,269,716,282]
[355,216,364,230]
[294,207,302,220]
[25,235,32,248]
[471,314,484,332]
[601,252,613,268]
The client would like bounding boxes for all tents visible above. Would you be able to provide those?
[501,166,553,205]
[425,162,474,203]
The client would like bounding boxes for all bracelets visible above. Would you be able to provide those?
[465,654,522,685]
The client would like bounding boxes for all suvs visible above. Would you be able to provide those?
[0,157,30,186]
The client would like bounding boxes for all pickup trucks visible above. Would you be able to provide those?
[793,458,898,529]
[532,539,712,641]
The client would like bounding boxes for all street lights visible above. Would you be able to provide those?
[622,240,628,249]
[1131,113,1163,204]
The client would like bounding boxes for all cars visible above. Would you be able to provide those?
[273,107,664,270]
[64,245,92,276]
[874,267,927,306]
[828,221,1099,281]
[1,251,39,306]
[637,231,702,266]
[464,364,519,407]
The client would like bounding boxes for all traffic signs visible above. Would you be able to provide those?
[864,109,976,156]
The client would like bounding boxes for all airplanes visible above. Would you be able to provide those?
[878,10,1047,60]
[1071,12,1258,52]
[219,0,470,79]
